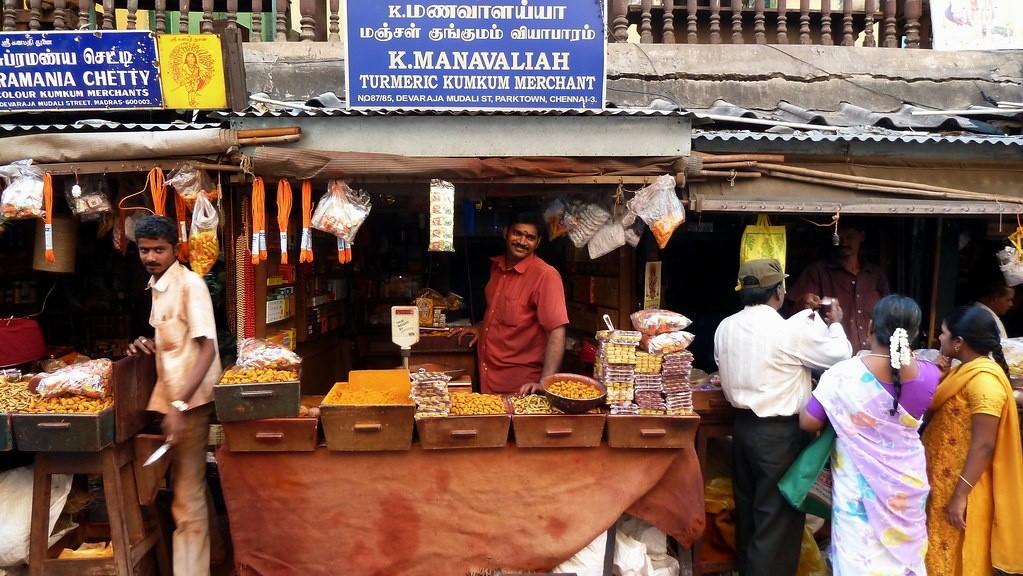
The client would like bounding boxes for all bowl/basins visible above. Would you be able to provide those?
[540,373,607,412]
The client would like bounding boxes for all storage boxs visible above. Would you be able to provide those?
[213,372,730,449]
[0,404,119,453]
[267,264,296,352]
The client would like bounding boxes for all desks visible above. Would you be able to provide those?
[215,445,699,576]
[693,407,734,576]
[25,442,171,576]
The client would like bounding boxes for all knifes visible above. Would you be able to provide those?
[143,436,170,466]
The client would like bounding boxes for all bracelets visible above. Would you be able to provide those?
[959,474,974,489]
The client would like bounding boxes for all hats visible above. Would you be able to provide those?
[739,259,790,289]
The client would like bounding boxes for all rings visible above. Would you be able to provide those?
[459,327,464,330]
[141,339,147,344]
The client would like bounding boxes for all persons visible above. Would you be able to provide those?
[127,215,223,576]
[714,258,853,576]
[974,282,1014,339]
[799,294,939,576]
[920,305,1023,576]
[446,213,569,395]
[790,222,882,355]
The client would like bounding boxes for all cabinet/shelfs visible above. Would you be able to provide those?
[266,281,295,326]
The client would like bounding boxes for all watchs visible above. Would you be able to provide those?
[170,400,189,412]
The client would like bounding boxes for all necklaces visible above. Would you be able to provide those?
[866,352,915,358]
